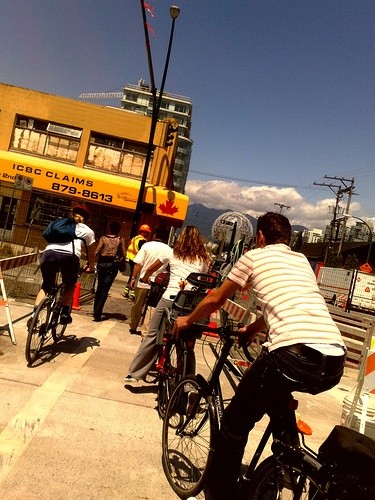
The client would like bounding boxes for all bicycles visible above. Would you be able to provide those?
[160,327,375,500]
[136,273,224,418]
[23,269,100,365]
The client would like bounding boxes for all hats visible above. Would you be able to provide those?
[73,206,91,219]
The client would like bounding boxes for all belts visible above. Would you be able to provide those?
[282,343,347,368]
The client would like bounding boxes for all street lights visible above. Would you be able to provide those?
[129,4,184,236]
[340,212,374,267]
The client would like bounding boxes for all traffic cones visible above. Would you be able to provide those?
[72,281,83,311]
[203,308,219,338]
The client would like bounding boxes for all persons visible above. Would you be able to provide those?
[173,211,346,500]
[124,223,210,383]
[83,222,126,321]
[26,205,95,327]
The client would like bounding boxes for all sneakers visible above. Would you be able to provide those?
[124,374,137,381]
[186,390,198,414]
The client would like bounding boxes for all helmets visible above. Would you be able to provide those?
[139,224,151,232]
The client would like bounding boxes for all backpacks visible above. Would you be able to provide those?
[42,217,78,243]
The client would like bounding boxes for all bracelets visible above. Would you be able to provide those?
[132,277,135,280]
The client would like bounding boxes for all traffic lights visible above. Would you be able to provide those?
[158,117,179,151]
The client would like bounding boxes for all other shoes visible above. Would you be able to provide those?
[27,318,33,326]
[122,292,129,298]
[129,328,141,335]
[128,295,135,302]
[94,314,108,321]
[60,313,72,324]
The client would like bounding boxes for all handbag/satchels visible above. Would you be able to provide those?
[115,243,125,272]
